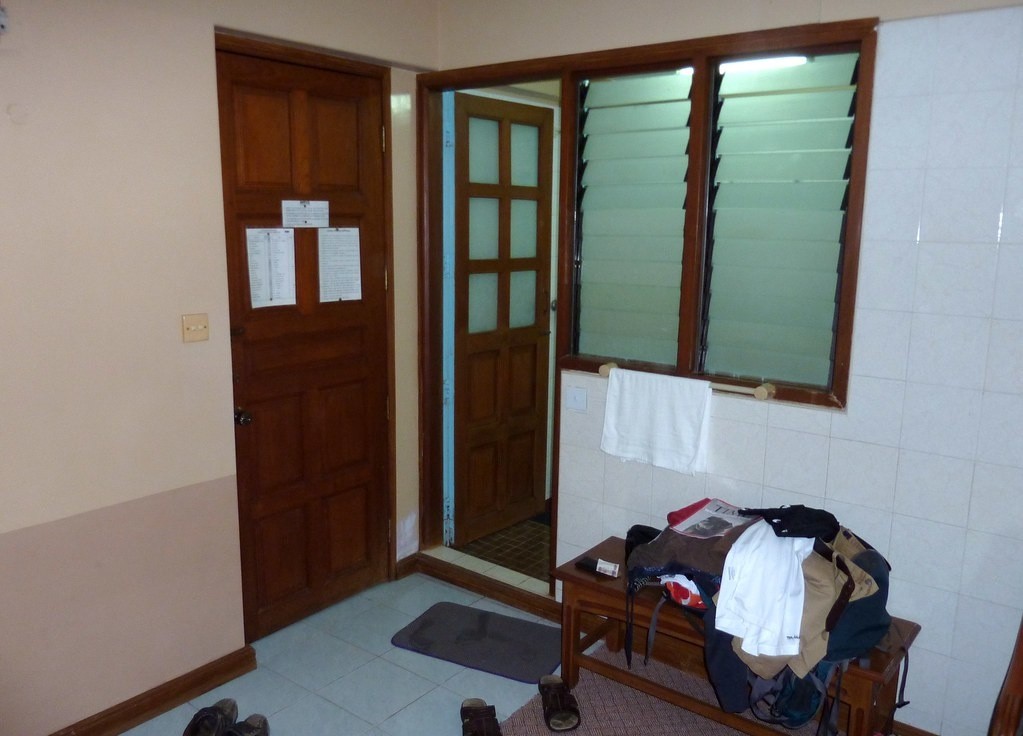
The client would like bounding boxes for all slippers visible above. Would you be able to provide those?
[460,698,502,736]
[538,674,581,732]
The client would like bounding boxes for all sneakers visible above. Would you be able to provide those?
[183,698,238,736]
[224,714,268,736]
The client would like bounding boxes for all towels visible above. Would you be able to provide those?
[599,367,712,477]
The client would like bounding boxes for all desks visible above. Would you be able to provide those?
[554,535,921,736]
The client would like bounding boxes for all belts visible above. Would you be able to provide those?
[813,537,855,632]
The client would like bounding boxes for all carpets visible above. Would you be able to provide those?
[500,643,896,736]
[392,601,563,684]
